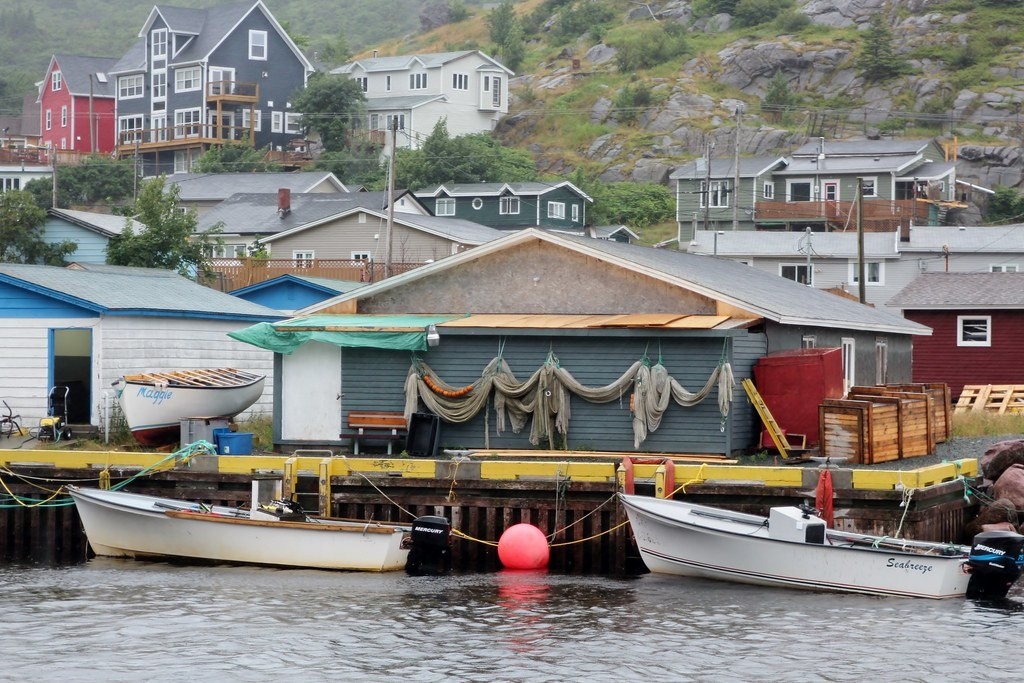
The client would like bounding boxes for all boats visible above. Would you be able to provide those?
[615,491,1024,602]
[65,483,451,573]
[111,368,267,447]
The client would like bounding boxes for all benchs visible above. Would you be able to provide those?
[339,411,407,456]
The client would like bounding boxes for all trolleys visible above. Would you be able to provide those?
[36,385,72,442]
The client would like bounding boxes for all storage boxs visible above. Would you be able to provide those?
[213,427,255,455]
[408,411,441,456]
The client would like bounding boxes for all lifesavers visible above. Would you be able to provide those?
[472,197,484,210]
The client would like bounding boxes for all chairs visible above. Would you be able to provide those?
[0,399,25,438]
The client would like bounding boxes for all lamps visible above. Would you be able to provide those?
[428,324,440,348]
